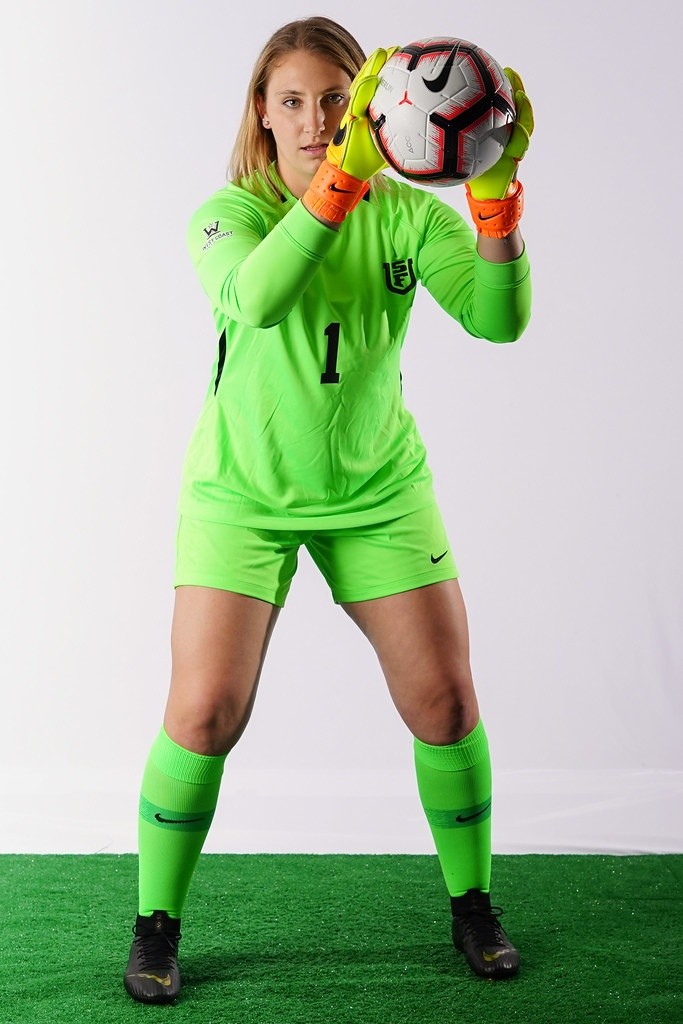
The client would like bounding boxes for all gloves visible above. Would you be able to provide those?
[465,67,535,202]
[325,45,390,182]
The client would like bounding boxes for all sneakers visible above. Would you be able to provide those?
[123,910,182,1004]
[450,888,520,980]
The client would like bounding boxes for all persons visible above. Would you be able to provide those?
[121,18,534,1004]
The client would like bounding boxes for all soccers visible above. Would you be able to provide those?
[368,36,518,191]
